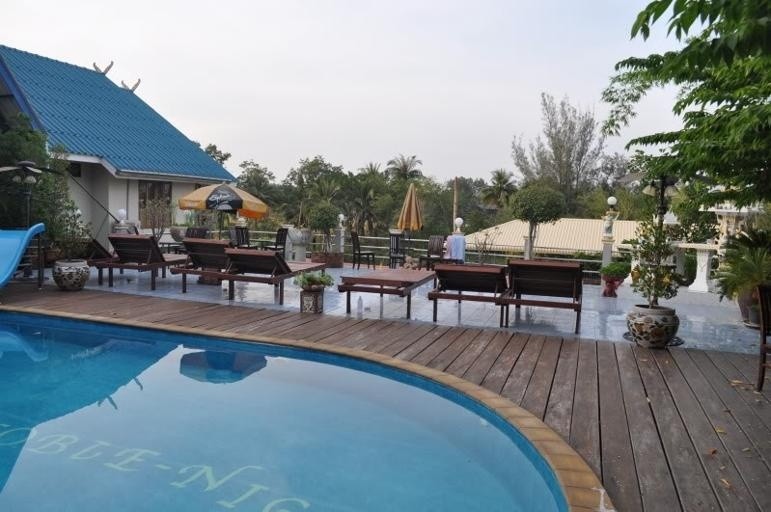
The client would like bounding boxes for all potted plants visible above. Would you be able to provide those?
[624,147,688,349]
[292,269,333,317]
[600,265,627,297]
[49,200,90,292]
[306,198,345,268]
[710,227,771,328]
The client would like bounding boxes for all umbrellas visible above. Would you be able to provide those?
[180,351,268,385]
[397,179,424,255]
[178,180,268,240]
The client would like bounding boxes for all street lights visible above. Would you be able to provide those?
[606,195,620,220]
[641,169,672,306]
[11,172,41,283]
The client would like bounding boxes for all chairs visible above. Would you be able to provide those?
[170,239,235,296]
[388,229,416,268]
[335,269,435,319]
[419,235,447,269]
[236,226,256,248]
[212,248,326,307]
[61,237,113,288]
[754,286,771,391]
[266,230,289,259]
[351,231,375,269]
[126,225,208,268]
[492,261,583,335]
[428,262,509,327]
[94,236,187,292]
